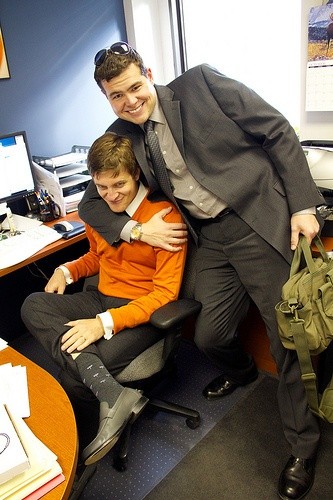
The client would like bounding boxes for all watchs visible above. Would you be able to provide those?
[130,222,144,244]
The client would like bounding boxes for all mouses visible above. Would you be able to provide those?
[54,221,74,231]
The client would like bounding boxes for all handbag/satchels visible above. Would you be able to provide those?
[275,232,333,423]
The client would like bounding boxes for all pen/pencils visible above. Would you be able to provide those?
[34,189,54,222]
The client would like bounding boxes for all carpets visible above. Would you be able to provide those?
[11,323,264,500]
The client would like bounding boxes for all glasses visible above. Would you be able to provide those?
[94,42,136,65]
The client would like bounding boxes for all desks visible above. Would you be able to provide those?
[0,346,78,500]
[0,210,87,278]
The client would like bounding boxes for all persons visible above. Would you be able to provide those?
[21,131,187,464]
[79,41,326,499]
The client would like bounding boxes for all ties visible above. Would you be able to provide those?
[144,119,198,248]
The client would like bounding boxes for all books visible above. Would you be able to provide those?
[0,362,65,500]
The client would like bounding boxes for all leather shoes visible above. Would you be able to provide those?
[202,374,258,400]
[80,387,150,465]
[277,454,317,500]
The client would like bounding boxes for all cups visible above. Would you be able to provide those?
[38,201,61,222]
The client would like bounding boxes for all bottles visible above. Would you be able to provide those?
[6,208,19,236]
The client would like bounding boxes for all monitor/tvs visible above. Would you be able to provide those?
[0,131,36,224]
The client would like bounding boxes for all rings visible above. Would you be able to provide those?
[80,336,86,341]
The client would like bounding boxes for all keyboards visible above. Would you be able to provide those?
[0,225,62,269]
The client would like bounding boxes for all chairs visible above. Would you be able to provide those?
[63,264,202,471]
